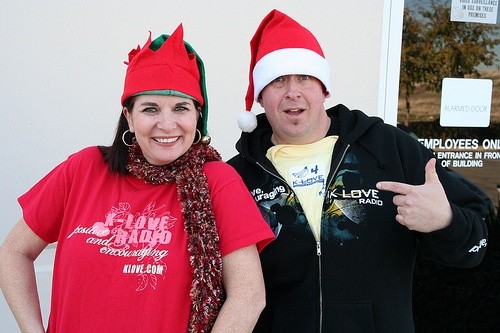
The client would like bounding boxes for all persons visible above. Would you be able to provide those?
[0,24,276,333]
[224,7,497,333]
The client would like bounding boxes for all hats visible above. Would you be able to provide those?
[120,23,212,144]
[237,9,332,133]
